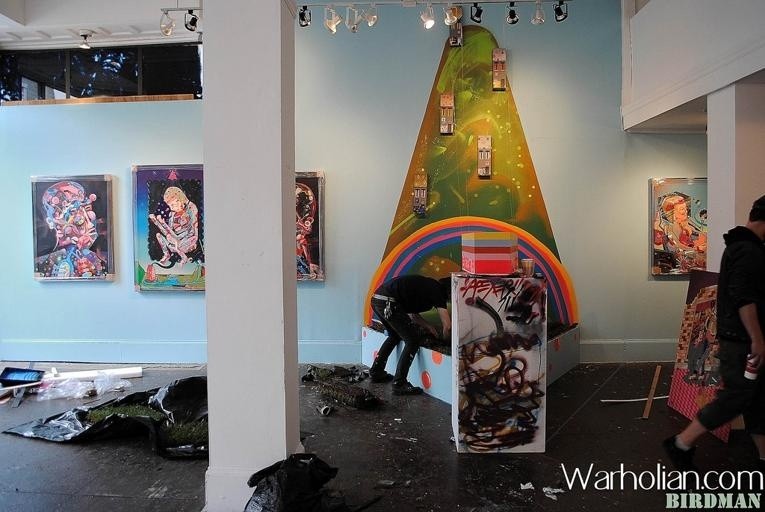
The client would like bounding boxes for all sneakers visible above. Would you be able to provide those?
[392,383,423,395]
[662,433,697,478]
[369,369,393,384]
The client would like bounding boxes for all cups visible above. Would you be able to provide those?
[521,258,535,277]
[744,353,760,379]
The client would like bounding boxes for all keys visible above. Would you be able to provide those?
[383,300,393,319]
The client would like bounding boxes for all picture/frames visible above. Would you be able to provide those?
[647,177,707,278]
[130,163,206,291]
[294,171,328,281]
[29,173,118,282]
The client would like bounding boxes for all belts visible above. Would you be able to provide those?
[372,293,395,302]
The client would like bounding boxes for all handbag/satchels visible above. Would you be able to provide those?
[244,452,338,512]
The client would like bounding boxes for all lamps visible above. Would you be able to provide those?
[158,0,572,48]
[77,30,94,50]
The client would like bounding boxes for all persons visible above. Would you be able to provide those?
[149,186,199,268]
[661,195,707,252]
[660,194,764,492]
[369,274,452,395]
